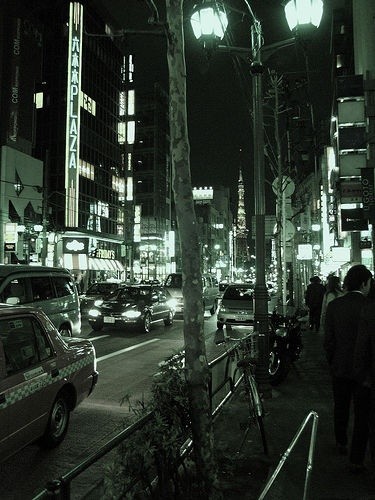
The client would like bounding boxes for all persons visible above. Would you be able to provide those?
[303,265,375,465]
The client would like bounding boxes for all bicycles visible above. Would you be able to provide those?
[215,329,271,456]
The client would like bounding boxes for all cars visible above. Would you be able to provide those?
[218,276,277,301]
[0,302,100,467]
[80,276,124,320]
[87,279,176,334]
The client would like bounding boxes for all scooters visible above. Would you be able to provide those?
[266,309,309,378]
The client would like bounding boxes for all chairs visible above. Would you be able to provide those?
[7,285,25,303]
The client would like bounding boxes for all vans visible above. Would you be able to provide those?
[164,271,220,320]
[0,262,83,338]
[216,282,256,330]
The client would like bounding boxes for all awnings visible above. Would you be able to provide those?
[64,254,123,272]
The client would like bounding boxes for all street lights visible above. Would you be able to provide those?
[188,0,326,386]
